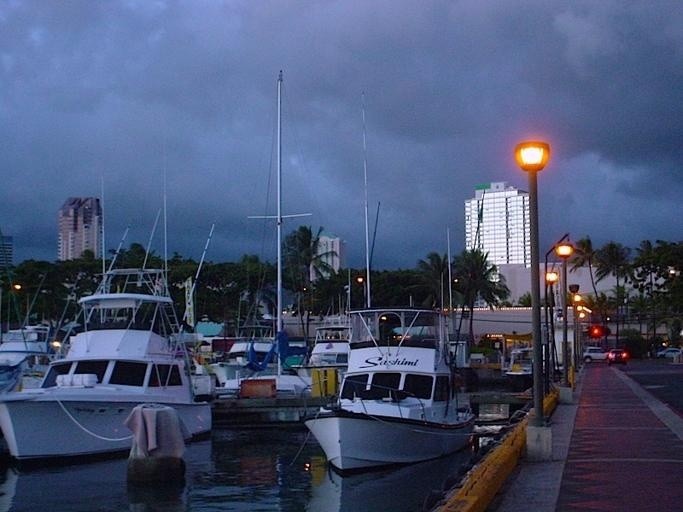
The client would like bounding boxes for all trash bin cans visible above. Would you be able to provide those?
[560,387,572,404]
[525,426,552,461]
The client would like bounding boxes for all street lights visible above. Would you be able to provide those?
[515,140,587,429]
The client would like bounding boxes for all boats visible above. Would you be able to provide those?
[327,442,475,507]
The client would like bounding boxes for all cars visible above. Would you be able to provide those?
[657,347,682,358]
[582,346,629,366]
